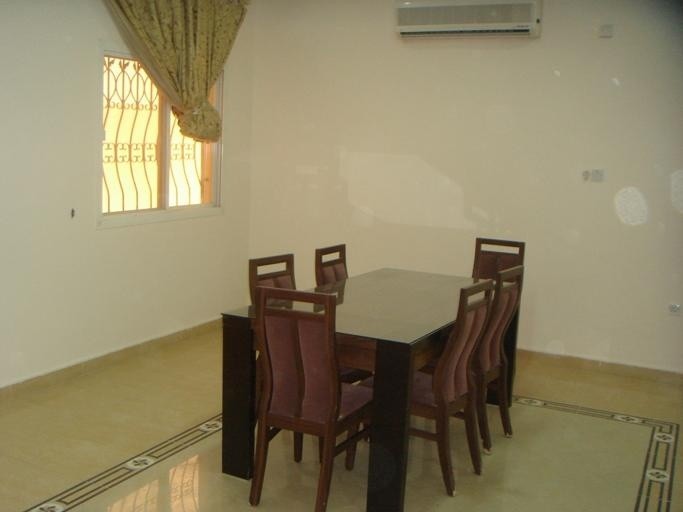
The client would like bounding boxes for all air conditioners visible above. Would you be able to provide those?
[395,1,543,38]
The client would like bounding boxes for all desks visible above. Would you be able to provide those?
[220,268,518,510]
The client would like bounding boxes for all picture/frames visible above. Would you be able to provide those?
[220,268,518,510]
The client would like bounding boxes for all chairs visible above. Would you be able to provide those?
[472,238,524,279]
[249,253,365,470]
[358,278,494,496]
[249,285,373,510]
[314,244,348,286]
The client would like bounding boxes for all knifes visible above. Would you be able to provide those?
[395,1,543,38]
